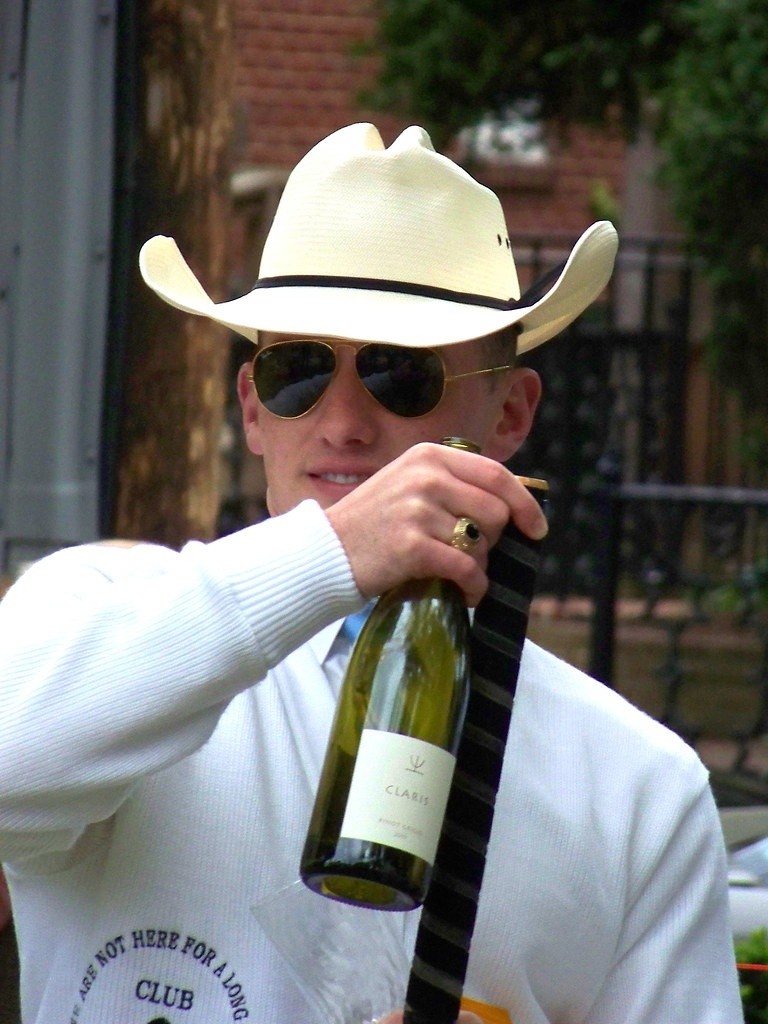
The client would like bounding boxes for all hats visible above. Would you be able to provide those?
[138,122,618,356]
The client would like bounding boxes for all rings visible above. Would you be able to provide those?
[452,519,480,553]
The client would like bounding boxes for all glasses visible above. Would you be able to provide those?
[246,340,510,420]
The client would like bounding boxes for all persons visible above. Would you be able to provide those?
[0,123,745,1024]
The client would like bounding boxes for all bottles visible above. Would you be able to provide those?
[296,434,482,912]
